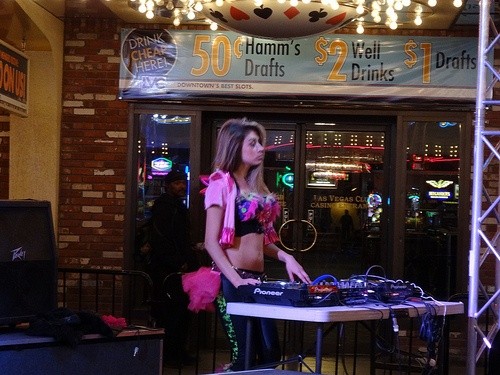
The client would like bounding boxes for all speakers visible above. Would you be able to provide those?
[0,200,57,327]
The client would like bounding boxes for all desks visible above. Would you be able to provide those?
[226,302,464,375]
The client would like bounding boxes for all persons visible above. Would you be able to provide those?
[145,168,198,367]
[340,209,354,241]
[202,118,313,372]
